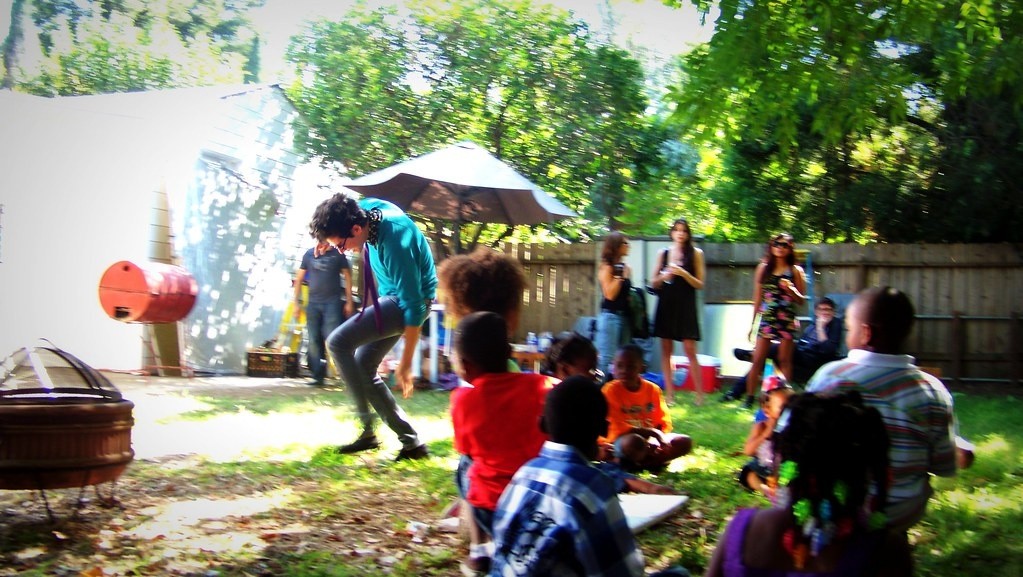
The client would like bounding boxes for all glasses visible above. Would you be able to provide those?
[770,241,790,248]
[335,236,347,250]
[622,241,627,245]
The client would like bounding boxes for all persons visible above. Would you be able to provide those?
[431,248,535,383]
[449,309,563,577]
[772,285,975,531]
[309,192,438,463]
[293,236,353,386]
[600,343,692,472]
[741,232,806,409]
[544,328,597,383]
[792,296,844,384]
[702,389,917,576]
[739,377,796,496]
[489,376,692,577]
[650,218,705,408]
[596,229,632,374]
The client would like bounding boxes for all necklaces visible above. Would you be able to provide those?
[368,206,383,247]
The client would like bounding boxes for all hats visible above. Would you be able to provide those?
[760,357,793,393]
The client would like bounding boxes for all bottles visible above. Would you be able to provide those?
[525,332,554,353]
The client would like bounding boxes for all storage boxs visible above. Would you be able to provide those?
[674,355,721,393]
[248,352,300,378]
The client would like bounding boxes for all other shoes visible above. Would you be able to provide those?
[396,443,428,462]
[460,555,494,577]
[339,436,380,454]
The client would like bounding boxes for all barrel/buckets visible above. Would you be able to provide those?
[100,263,197,324]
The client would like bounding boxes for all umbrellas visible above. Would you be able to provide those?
[343,139,581,358]
